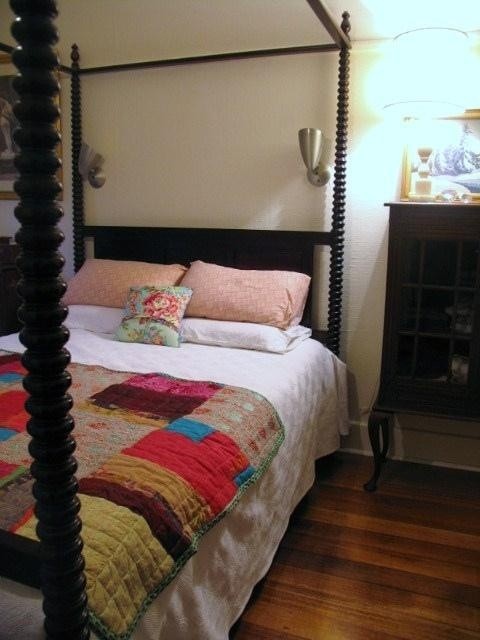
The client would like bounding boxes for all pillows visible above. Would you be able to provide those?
[56,257,313,349]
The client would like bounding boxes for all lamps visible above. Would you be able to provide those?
[298,127,331,187]
[74,141,107,190]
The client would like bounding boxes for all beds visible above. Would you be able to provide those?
[0,223,346,640]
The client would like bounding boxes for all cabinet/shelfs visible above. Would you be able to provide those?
[362,195,480,492]
